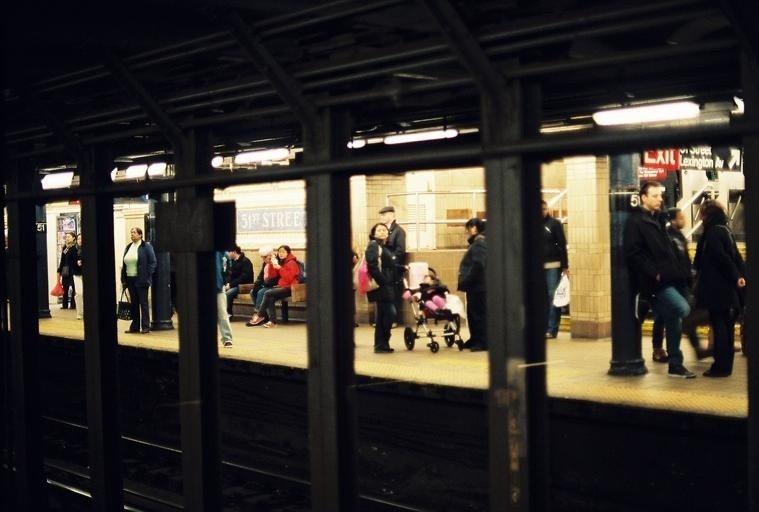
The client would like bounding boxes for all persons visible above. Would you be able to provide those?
[542,200,568,339]
[120,227,157,333]
[352,206,488,353]
[57,232,77,309]
[625,182,746,378]
[216,244,301,348]
[72,234,84,320]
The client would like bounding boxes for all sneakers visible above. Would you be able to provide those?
[545,332,554,338]
[653,350,669,362]
[703,364,731,377]
[669,368,696,379]
[697,350,713,359]
[264,321,277,328]
[463,340,488,352]
[635,293,648,322]
[223,340,233,347]
[248,309,265,324]
[375,348,394,352]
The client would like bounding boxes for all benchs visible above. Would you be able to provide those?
[218,253,306,327]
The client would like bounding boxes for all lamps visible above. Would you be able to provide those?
[383,124,459,151]
[40,143,301,189]
[591,97,703,131]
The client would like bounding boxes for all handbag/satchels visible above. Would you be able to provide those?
[353,256,381,294]
[118,289,133,320]
[51,283,65,295]
[553,273,570,307]
[668,268,687,288]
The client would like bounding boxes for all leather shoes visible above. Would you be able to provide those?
[141,329,149,333]
[125,330,140,333]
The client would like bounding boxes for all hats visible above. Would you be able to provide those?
[379,206,394,213]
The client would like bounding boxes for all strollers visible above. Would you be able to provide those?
[398,262,466,355]
